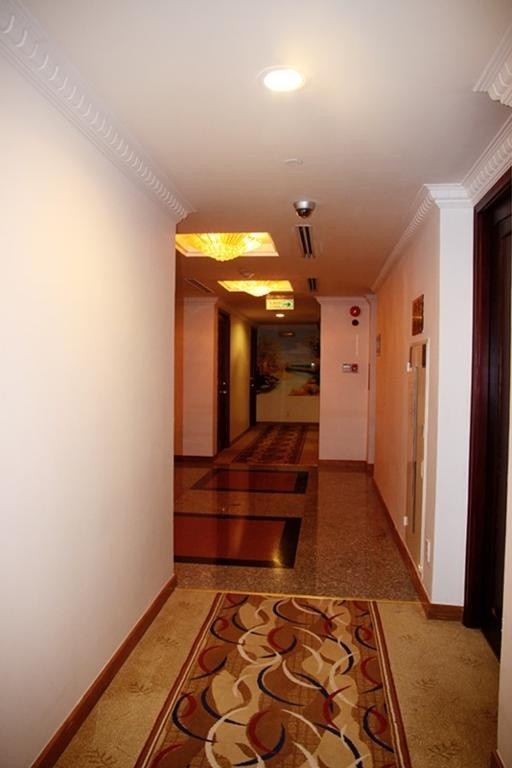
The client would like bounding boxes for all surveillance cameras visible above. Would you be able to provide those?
[291,201,315,221]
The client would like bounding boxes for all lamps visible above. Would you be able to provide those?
[173,231,280,261]
[217,280,293,300]
